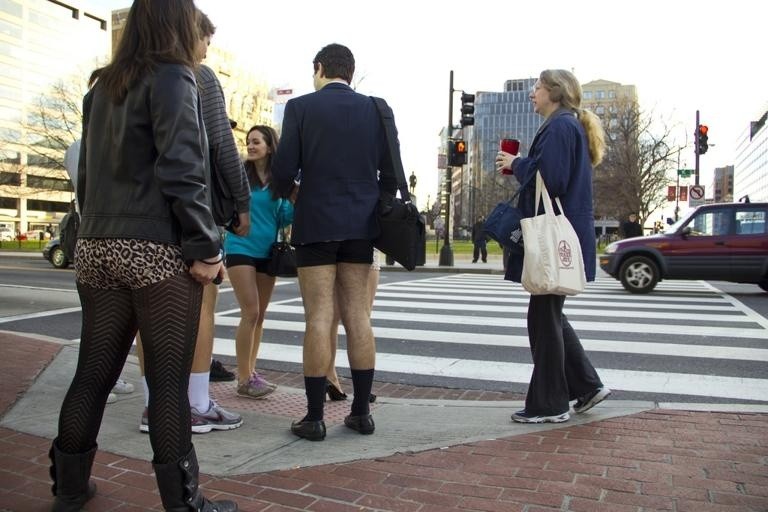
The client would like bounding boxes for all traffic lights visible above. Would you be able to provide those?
[461,91,475,127]
[453,141,468,164]
[697,125,708,154]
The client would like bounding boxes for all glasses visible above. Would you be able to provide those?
[532,84,553,93]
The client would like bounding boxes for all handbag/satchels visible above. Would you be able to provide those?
[372,192,424,271]
[483,203,524,257]
[59,201,78,261]
[268,241,298,278]
[210,149,235,224]
[521,215,586,295]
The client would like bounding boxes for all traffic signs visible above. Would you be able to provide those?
[681,170,690,178]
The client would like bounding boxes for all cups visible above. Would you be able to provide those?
[497,138,521,176]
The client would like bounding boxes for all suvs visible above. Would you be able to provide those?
[600,195,766,295]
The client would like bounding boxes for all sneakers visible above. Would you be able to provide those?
[512,410,569,423]
[573,388,610,412]
[105,359,375,440]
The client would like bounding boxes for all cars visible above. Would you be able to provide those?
[42,237,74,268]
[736,218,766,235]
[0,226,52,242]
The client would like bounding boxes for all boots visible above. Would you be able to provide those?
[49,437,95,512]
[153,446,238,512]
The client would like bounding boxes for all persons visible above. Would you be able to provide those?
[687,211,729,237]
[470,215,491,263]
[223,125,295,399]
[47,1,251,512]
[622,212,643,237]
[494,70,611,424]
[320,242,382,405]
[273,45,402,438]
